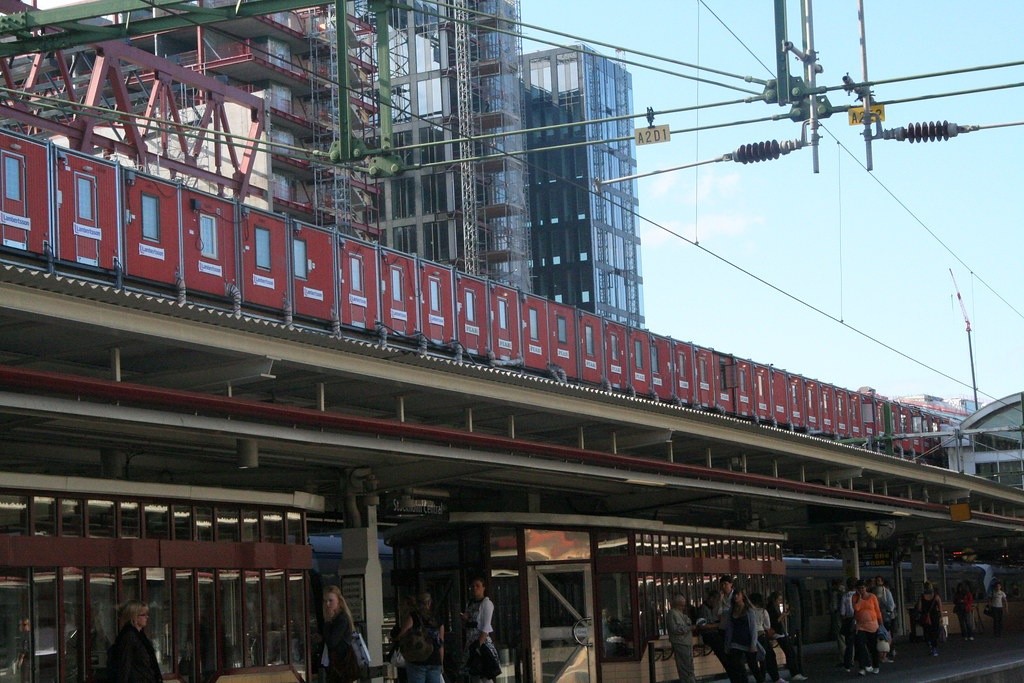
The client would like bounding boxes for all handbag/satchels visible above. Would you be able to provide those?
[919,611,932,626]
[391,647,406,669]
[877,636,890,652]
[399,628,433,663]
[320,632,371,668]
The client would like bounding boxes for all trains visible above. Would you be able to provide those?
[309,535,1024,668]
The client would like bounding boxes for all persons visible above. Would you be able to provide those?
[321,585,363,683]
[391,590,448,683]
[828,575,898,676]
[16,617,33,683]
[988,581,1008,640]
[110,599,164,683]
[917,582,944,657]
[696,575,810,683]
[667,593,698,683]
[460,577,503,683]
[952,583,976,641]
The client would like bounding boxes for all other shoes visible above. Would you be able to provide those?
[775,677,789,683]
[792,673,809,681]
[882,658,894,663]
[858,669,866,676]
[865,665,874,672]
[839,666,851,672]
[872,667,879,673]
[929,647,938,657]
[965,636,974,641]
